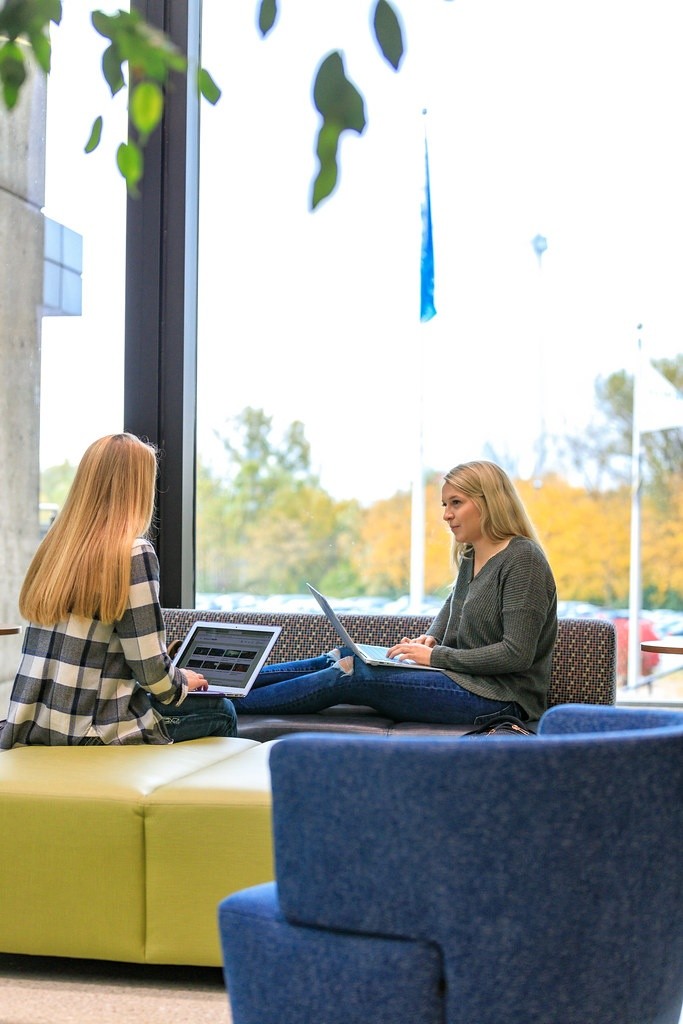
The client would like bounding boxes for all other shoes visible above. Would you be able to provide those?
[167,639,183,660]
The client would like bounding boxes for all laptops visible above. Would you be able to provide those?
[172,620,282,698]
[306,583,445,671]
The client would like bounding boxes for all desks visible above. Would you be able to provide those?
[641,640,682,656]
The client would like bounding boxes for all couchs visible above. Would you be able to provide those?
[218,703,683,1024]
[161,608,618,744]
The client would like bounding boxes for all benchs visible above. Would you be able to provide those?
[1,736,282,984]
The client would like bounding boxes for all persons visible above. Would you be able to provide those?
[170,460,557,724]
[0,434,237,747]
